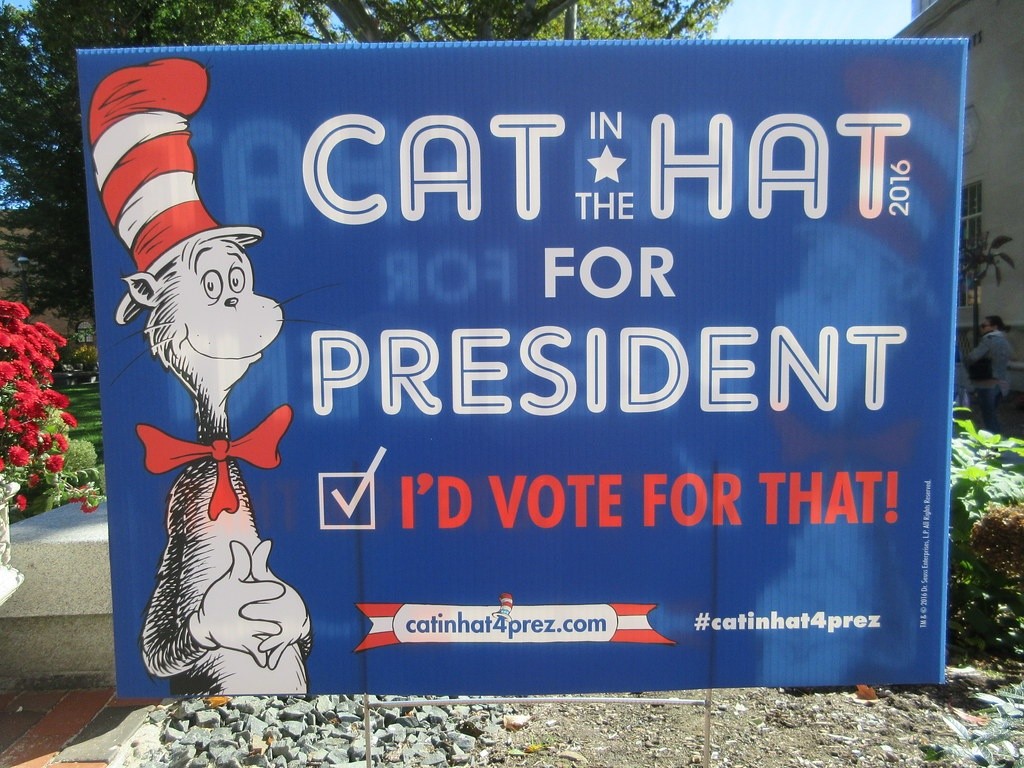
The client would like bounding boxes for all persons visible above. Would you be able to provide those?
[964,316,1013,435]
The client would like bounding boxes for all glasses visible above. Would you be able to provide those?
[978,324,990,328]
